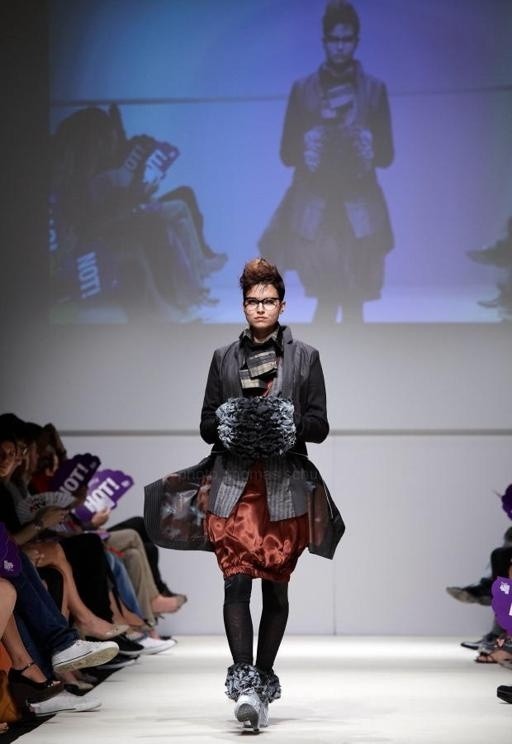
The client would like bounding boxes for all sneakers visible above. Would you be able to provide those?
[51,588,187,688]
[30,690,102,717]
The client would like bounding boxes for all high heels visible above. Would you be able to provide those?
[8,662,63,700]
[235,692,269,731]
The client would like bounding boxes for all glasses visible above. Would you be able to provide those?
[243,297,279,311]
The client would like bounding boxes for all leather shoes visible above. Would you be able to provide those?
[461,634,512,663]
[497,685,511,703]
[447,585,492,605]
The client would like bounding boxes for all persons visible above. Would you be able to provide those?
[442,480,512,704]
[267,0,396,324]
[0,412,186,744]
[455,212,511,321]
[196,261,330,738]
[49,97,225,321]
